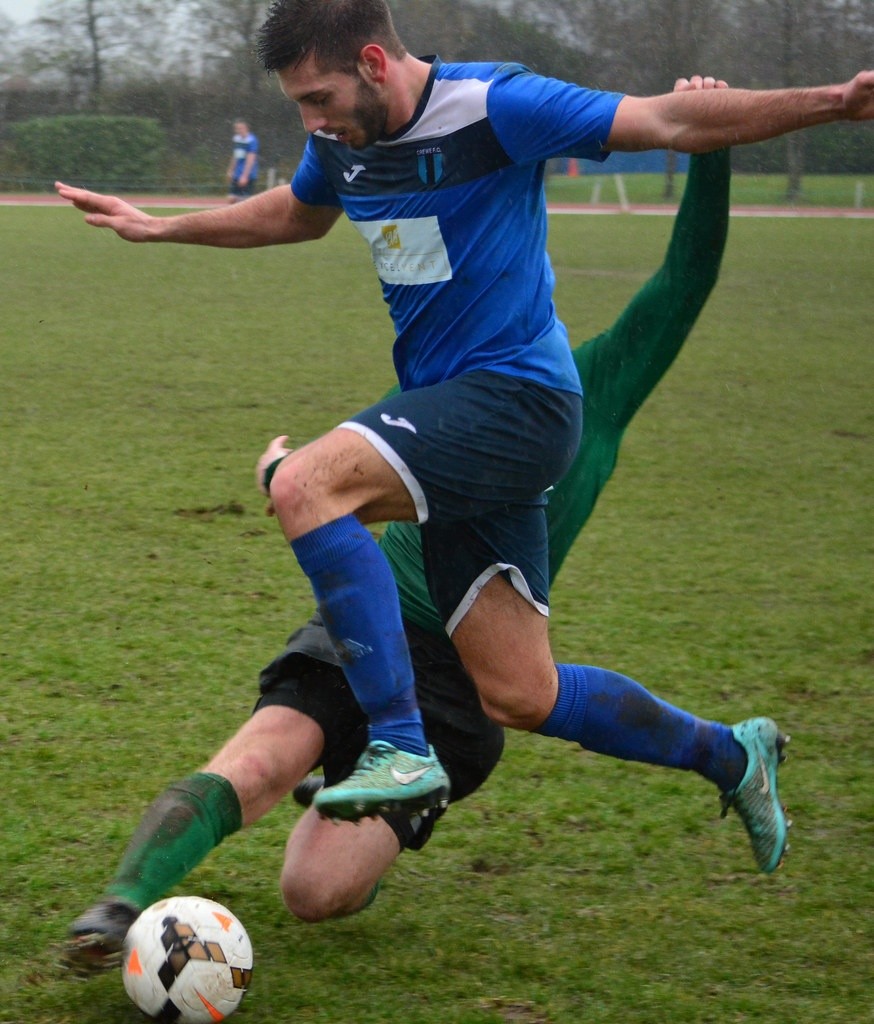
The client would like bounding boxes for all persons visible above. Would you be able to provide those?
[49,0,874,871]
[57,74,730,976]
[226,118,259,205]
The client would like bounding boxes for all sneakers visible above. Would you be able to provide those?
[717,716,793,873]
[310,740,449,822]
[290,774,322,804]
[63,898,143,976]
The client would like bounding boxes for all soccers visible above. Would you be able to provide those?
[122,894,255,1024]
